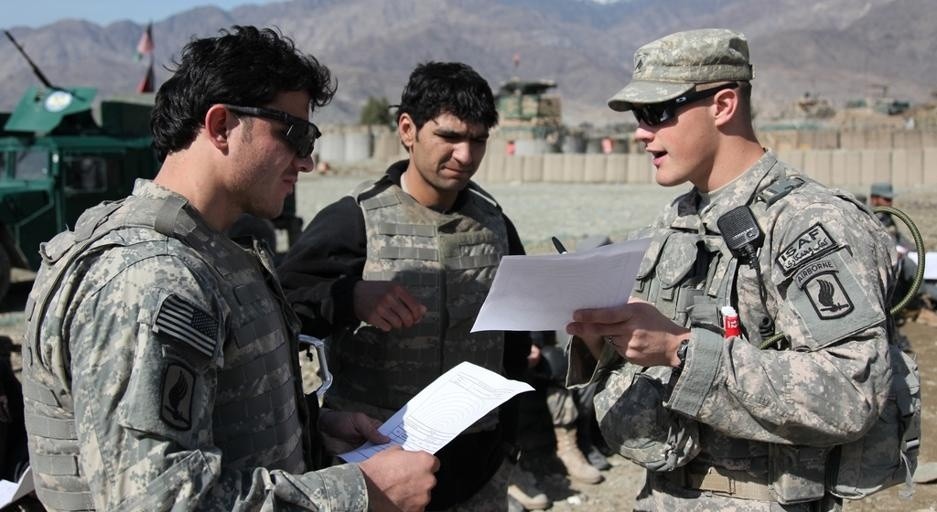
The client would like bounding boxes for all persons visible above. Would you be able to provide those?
[871,182,937,312]
[279,58,533,512]
[490,322,601,512]
[573,371,617,471]
[16,20,443,511]
[567,26,922,512]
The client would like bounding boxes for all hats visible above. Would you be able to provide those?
[607,28,757,112]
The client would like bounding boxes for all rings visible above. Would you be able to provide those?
[606,334,618,346]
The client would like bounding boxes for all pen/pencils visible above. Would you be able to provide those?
[551,236,568,255]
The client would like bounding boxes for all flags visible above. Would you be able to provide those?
[132,21,153,64]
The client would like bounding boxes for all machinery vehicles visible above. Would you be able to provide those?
[1,30,302,267]
[493,82,560,139]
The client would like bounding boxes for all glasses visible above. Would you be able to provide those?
[631,81,743,130]
[205,98,324,157]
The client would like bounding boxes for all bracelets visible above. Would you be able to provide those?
[672,336,690,377]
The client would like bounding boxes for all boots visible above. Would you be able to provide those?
[490,421,609,509]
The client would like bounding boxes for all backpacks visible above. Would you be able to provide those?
[20,196,200,511]
[773,190,921,500]
